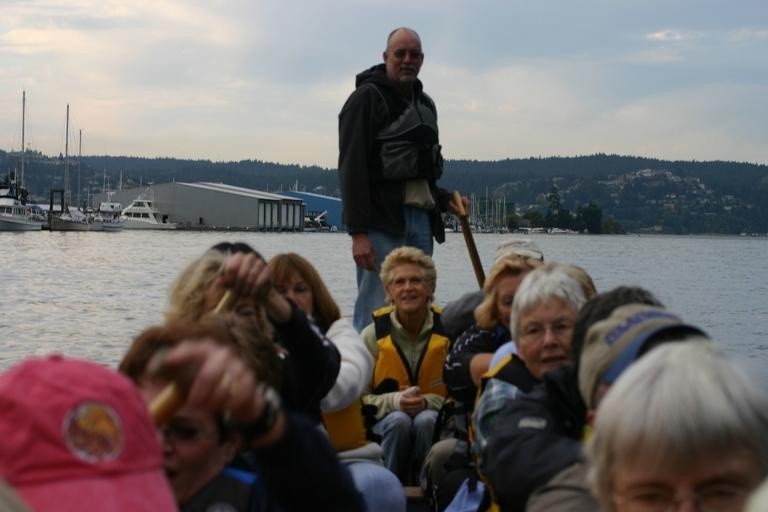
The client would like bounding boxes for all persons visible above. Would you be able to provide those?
[2,232,768,512]
[337,26,471,331]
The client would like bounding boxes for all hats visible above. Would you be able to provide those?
[0,355,177,512]
[578,303,712,408]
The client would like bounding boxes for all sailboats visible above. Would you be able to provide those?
[443,185,507,233]
[0,89,177,233]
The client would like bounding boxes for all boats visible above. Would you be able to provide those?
[514,226,578,235]
[302,209,338,232]
